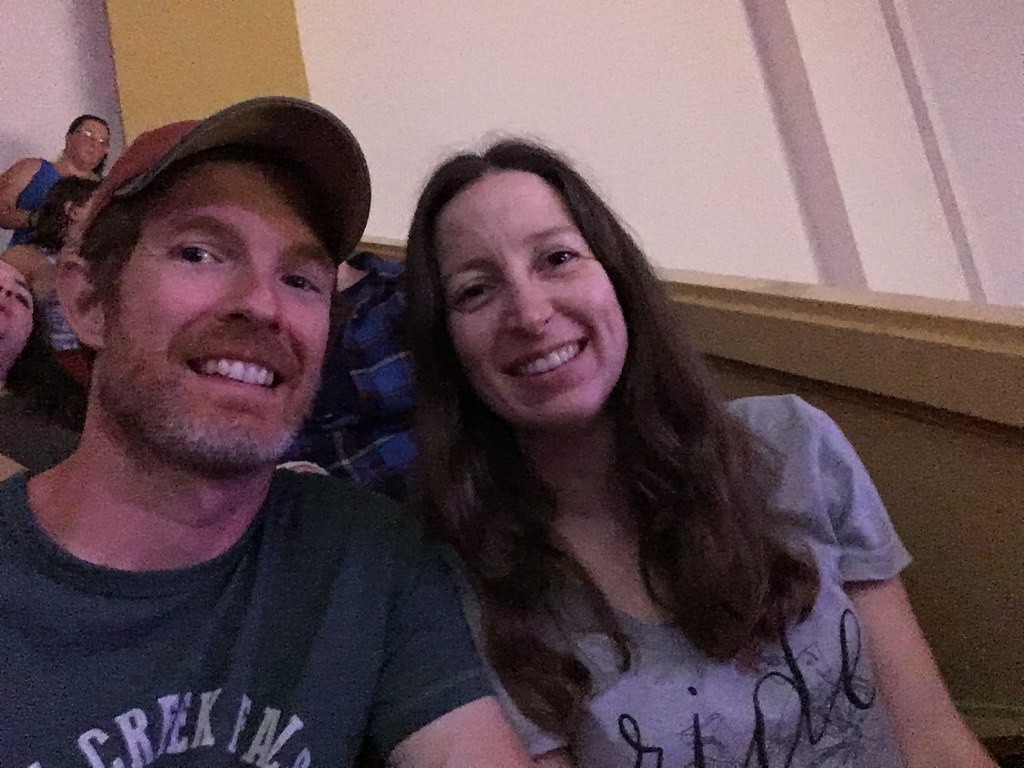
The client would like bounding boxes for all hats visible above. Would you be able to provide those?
[76,95,372,265]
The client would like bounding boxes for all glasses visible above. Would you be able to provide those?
[72,129,109,146]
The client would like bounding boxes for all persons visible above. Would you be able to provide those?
[0,113,112,249]
[0,93,537,768]
[0,175,111,367]
[397,139,1005,768]
[0,257,43,488]
